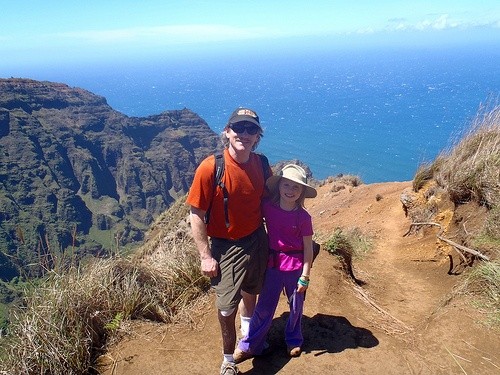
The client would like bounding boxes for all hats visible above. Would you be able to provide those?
[265,164,317,199]
[227,108,260,126]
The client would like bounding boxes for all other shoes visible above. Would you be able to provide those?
[221,361,236,375]
[288,346,302,354]
[235,348,253,362]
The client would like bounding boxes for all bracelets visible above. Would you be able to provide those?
[298,275,310,286]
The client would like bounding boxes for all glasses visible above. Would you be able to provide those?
[233,126,256,134]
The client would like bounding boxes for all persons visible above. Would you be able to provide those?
[185,108,275,375]
[232,164,314,363]
